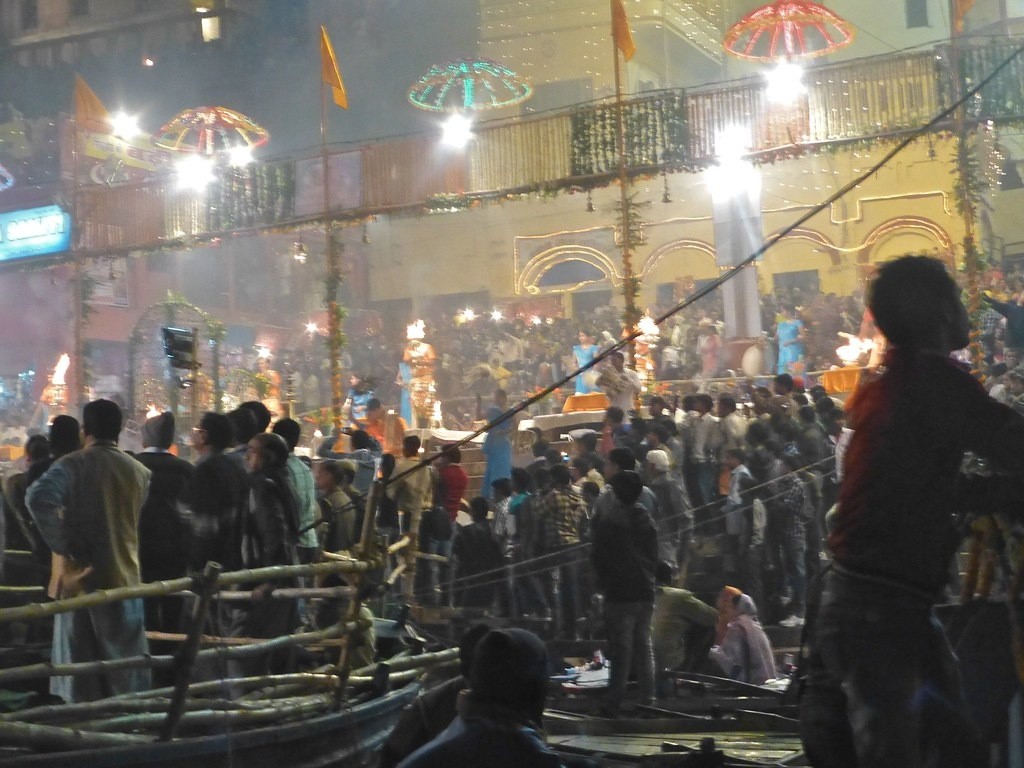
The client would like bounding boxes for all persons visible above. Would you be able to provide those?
[0,253,1024,768]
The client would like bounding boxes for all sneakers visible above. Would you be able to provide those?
[779,614,805,627]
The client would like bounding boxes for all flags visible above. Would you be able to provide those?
[610,0,636,63]
[320,26,347,110]
[73,74,114,134]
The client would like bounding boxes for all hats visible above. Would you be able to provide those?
[468,628,550,717]
[141,412,174,447]
[569,429,597,451]
[646,450,670,473]
[793,376,806,387]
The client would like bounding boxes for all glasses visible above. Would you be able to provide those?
[191,426,208,434]
